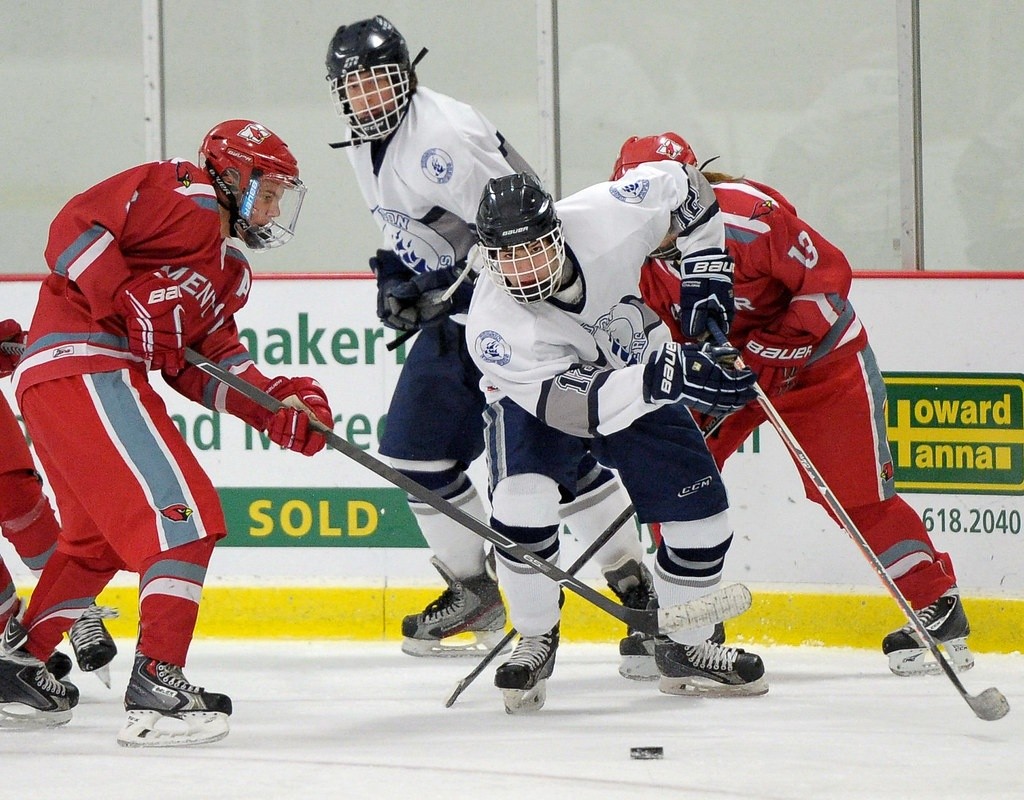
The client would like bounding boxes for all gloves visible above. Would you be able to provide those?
[110,269,186,376]
[369,249,480,331]
[680,247,736,339]
[741,327,821,408]
[258,376,334,457]
[650,341,759,416]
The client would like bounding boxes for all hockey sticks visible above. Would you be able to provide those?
[443,412,732,708]
[707,315,1012,723]
[181,343,753,635]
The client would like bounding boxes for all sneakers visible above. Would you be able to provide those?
[117,621,233,747]
[882,593,974,677]
[401,555,513,657]
[67,601,118,689]
[603,559,725,681]
[494,619,565,714]
[0,615,79,733]
[654,635,769,697]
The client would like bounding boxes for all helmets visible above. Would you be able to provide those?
[476,170,566,303]
[201,119,307,250]
[613,133,697,260]
[326,14,428,148]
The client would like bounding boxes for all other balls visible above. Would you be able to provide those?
[630,745,664,760]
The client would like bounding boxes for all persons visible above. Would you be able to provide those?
[323,16,662,682]
[0,120,334,749]
[0,319,116,696]
[465,161,769,715]
[610,132,976,676]
